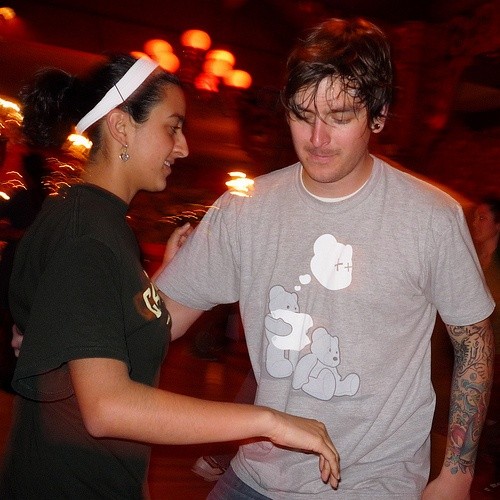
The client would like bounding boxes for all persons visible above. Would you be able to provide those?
[0,56,340,500]
[153,17,496,500]
[472,194,500,500]
[0,135,257,481]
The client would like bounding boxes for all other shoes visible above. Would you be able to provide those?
[191,348,218,362]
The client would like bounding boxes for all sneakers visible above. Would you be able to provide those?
[192,455,226,483]
